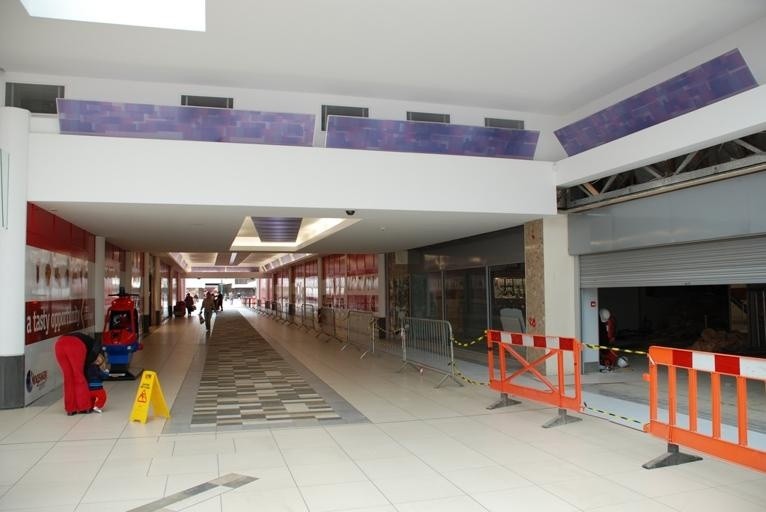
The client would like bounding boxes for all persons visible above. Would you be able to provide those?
[184,293,194,317]
[54,329,110,416]
[199,291,223,335]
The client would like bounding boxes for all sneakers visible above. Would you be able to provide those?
[92,406,101,413]
